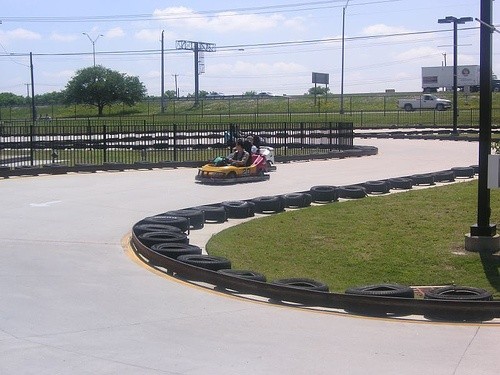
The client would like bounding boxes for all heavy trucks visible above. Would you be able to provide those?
[421,64,500,92]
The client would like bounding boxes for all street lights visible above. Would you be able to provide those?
[437,16,473,136]
[83,32,104,68]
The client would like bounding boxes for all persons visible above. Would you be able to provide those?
[247,136,258,154]
[226,139,249,166]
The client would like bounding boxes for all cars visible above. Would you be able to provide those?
[259,92,275,96]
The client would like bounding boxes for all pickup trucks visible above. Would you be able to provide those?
[396,94,451,111]
